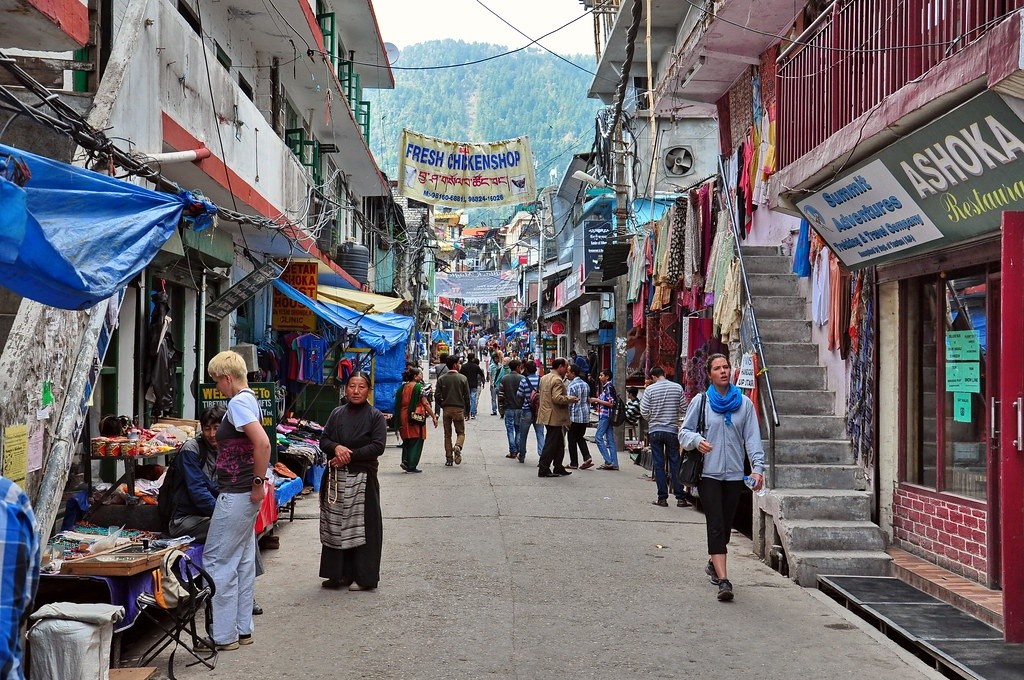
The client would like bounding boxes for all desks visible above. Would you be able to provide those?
[39,542,206,668]
[273,476,303,522]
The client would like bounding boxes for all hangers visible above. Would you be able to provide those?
[308,329,319,338]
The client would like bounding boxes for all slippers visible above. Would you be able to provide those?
[579,463,594,469]
[606,466,618,470]
[596,464,612,470]
[564,464,577,469]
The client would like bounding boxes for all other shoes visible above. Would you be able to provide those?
[445,461,453,466]
[677,500,692,507]
[239,634,253,645]
[517,453,524,463]
[252,605,263,614]
[349,581,373,590]
[407,468,422,472]
[454,446,461,465]
[193,635,240,651]
[653,499,668,507]
[505,452,517,458]
[322,579,352,588]
[400,463,407,470]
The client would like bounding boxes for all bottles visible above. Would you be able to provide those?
[742,476,767,497]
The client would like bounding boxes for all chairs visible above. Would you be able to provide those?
[625,419,638,441]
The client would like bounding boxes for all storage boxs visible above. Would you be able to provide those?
[60,542,190,576]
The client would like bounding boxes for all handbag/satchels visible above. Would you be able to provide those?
[676,391,706,487]
[411,411,426,424]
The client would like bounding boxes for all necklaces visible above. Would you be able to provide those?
[625,387,641,426]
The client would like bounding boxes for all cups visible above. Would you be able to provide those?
[40,544,64,568]
[108,526,119,536]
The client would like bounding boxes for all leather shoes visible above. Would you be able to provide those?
[553,468,572,475]
[538,469,559,477]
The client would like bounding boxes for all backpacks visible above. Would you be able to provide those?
[523,376,541,420]
[152,549,217,611]
[604,384,625,426]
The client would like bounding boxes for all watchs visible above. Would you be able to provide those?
[252,476,268,486]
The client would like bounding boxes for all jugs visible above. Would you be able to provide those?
[127,429,141,439]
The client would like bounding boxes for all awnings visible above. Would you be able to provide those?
[543,309,565,320]
[504,320,527,335]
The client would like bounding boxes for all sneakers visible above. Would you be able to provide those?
[705,558,719,585]
[717,578,734,599]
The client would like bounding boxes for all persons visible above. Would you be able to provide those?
[158,406,264,615]
[677,353,765,603]
[537,357,579,478]
[435,355,471,466]
[193,350,271,652]
[588,370,620,471]
[565,364,594,470]
[318,370,387,591]
[0,476,41,680]
[435,329,534,419]
[397,369,438,474]
[640,365,689,507]
[516,361,545,468]
[406,362,414,373]
[568,351,599,410]
[499,360,525,458]
